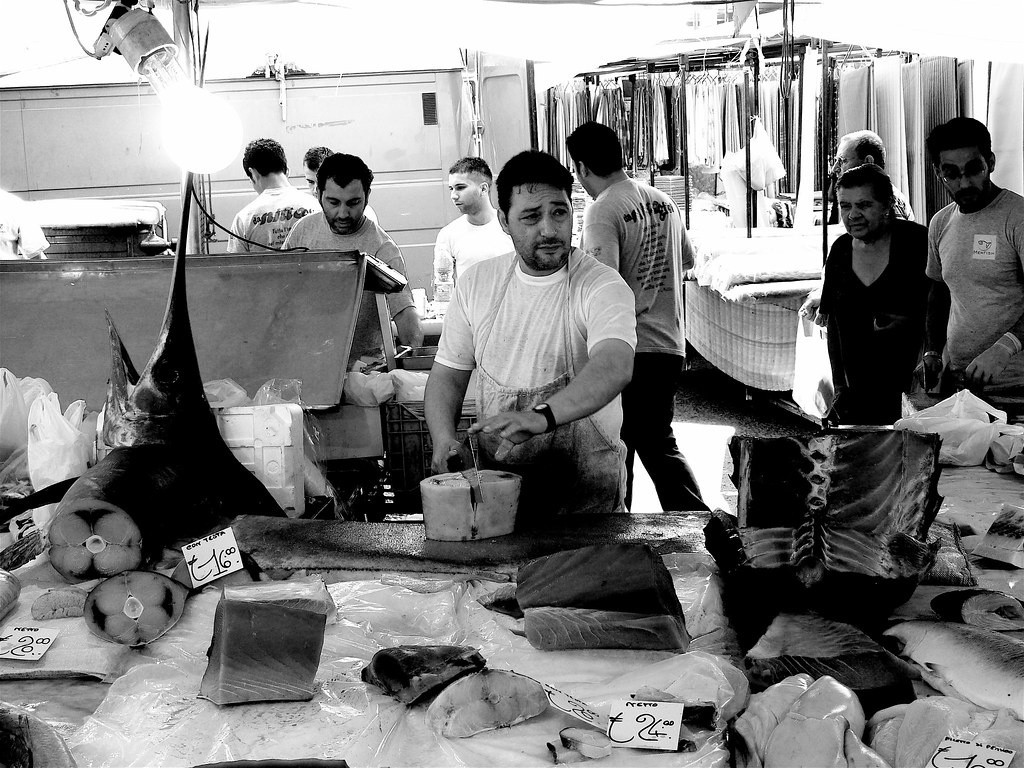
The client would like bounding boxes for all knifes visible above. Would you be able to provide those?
[448,435,485,506]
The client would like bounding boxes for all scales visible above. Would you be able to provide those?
[363,253,439,373]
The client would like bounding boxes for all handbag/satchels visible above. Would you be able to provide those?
[792,311,835,422]
[895,389,1007,468]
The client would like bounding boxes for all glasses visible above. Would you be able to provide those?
[834,156,866,167]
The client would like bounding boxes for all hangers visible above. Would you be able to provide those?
[553,42,875,93]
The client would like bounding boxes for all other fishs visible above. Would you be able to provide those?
[27,180,334,706]
[367,422,1023,767]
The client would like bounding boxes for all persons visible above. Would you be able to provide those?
[797,130,917,325]
[304,147,380,226]
[910,115,1024,425]
[430,154,515,302]
[564,119,710,511]
[822,163,951,430]
[276,152,424,358]
[423,145,637,518]
[768,202,793,227]
[225,136,320,256]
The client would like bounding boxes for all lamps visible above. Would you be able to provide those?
[108,8,247,176]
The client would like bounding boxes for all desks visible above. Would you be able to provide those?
[390,300,445,337]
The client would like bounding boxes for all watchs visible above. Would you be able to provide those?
[533,403,557,434]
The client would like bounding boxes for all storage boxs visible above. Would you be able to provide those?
[213,403,305,519]
[399,346,437,371]
[305,404,384,462]
[386,400,479,498]
[792,312,834,419]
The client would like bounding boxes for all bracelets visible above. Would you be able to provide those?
[1004,330,1022,353]
[924,351,938,356]
